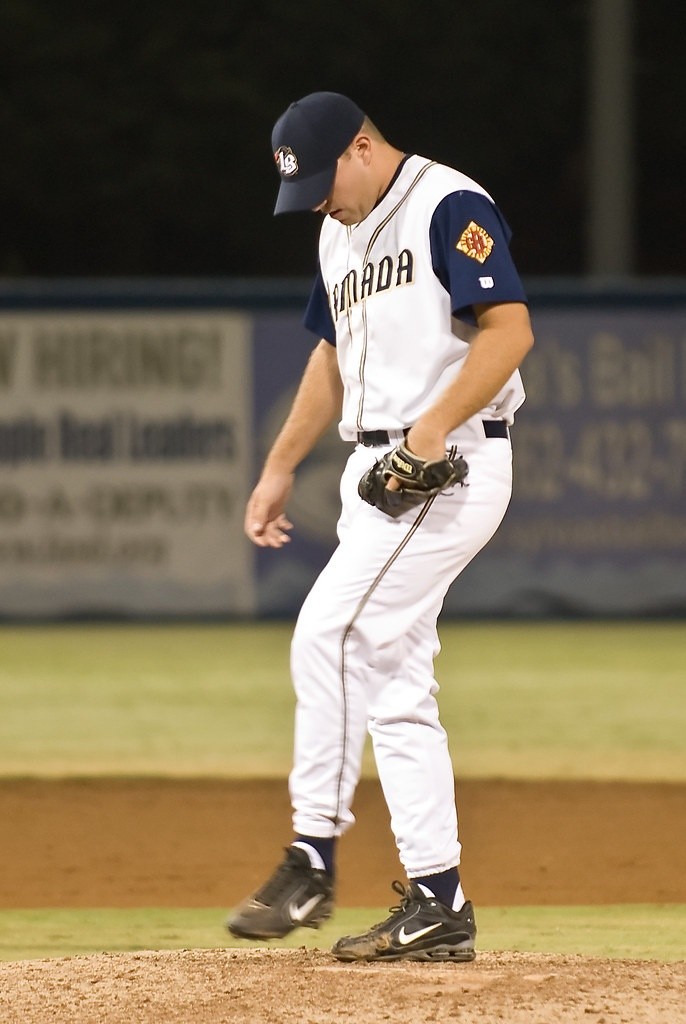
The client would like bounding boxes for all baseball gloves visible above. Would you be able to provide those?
[356,443,471,519]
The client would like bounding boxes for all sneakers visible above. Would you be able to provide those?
[331,880,478,962]
[228,846,335,940]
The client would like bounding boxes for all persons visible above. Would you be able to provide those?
[224,91,537,963]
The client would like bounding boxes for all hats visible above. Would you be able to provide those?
[272,92,365,215]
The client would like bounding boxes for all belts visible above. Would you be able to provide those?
[356,421,508,445]
